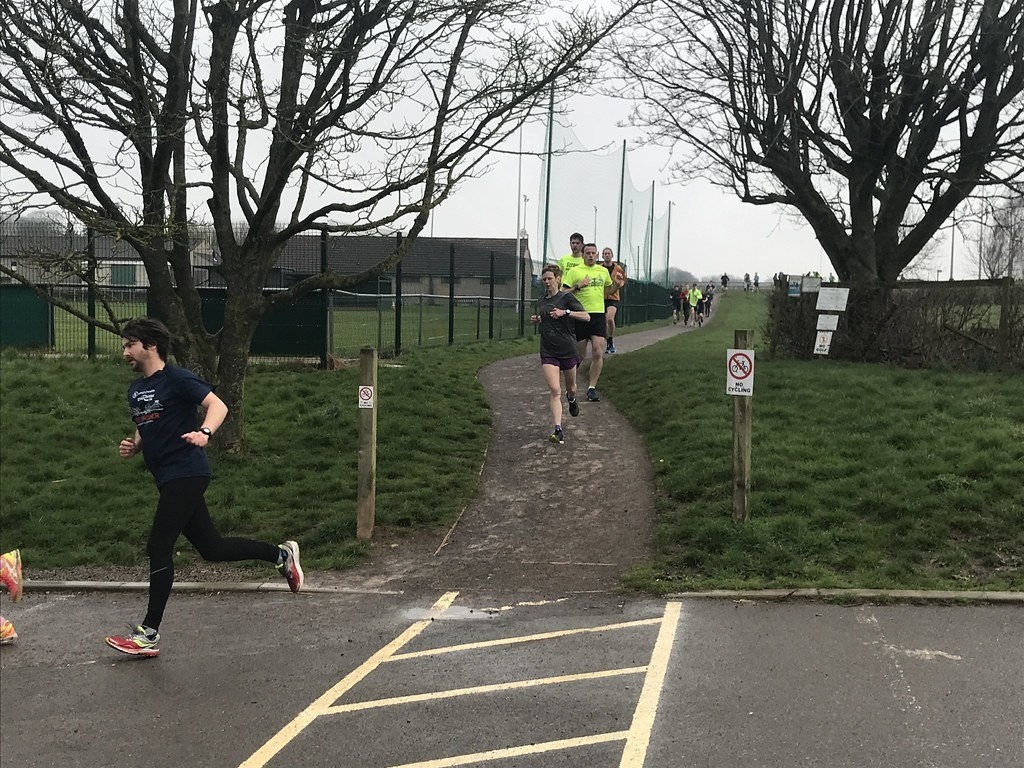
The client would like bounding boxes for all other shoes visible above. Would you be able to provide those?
[672,315,704,327]
[604,346,616,354]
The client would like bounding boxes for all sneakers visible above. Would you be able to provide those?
[0,548,22,603]
[586,388,601,402]
[103,623,161,657]
[547,428,564,444]
[274,540,304,593]
[0,616,18,644]
[566,393,579,417]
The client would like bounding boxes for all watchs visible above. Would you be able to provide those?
[198,427,212,440]
[566,309,571,317]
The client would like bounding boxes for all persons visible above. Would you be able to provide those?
[0,549,22,643]
[753,272,759,287]
[670,281,716,327]
[531,265,590,444]
[599,247,625,353]
[829,273,835,283]
[806,271,819,278]
[773,272,783,286]
[557,232,584,291]
[562,243,623,401]
[721,273,730,293]
[744,272,750,290]
[106,319,304,657]
